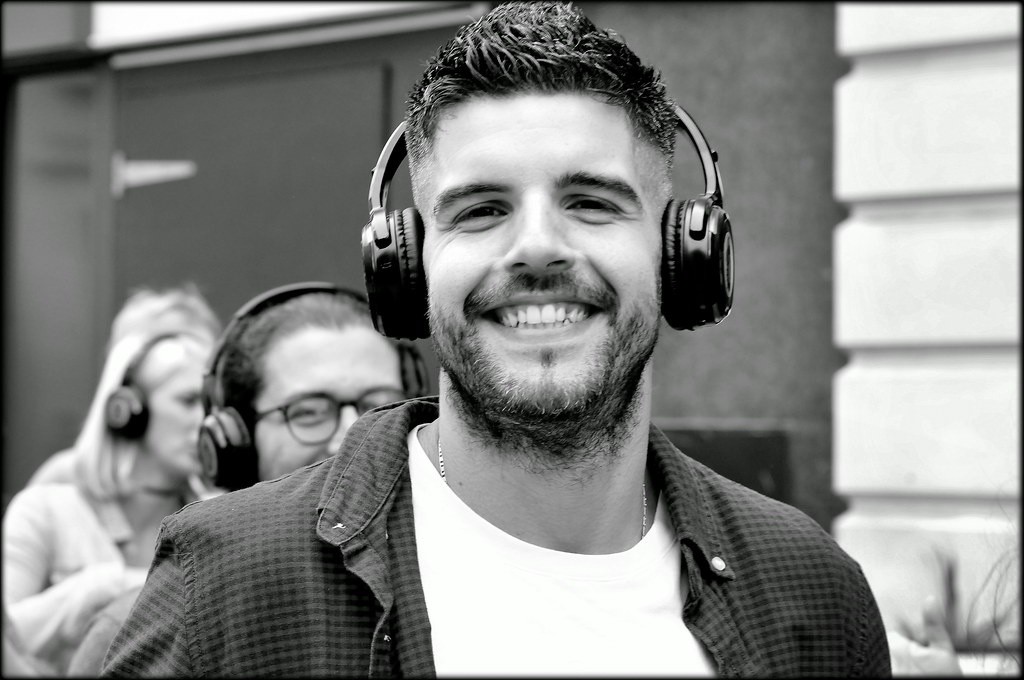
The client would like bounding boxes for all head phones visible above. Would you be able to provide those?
[104,326,210,444]
[357,86,736,345]
[196,279,429,494]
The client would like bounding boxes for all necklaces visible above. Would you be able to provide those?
[439,438,646,539]
[139,485,177,497]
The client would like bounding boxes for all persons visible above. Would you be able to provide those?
[65,281,431,678]
[4,327,225,677]
[97,5,891,680]
[108,291,223,355]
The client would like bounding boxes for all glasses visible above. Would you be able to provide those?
[250,386,405,446]
